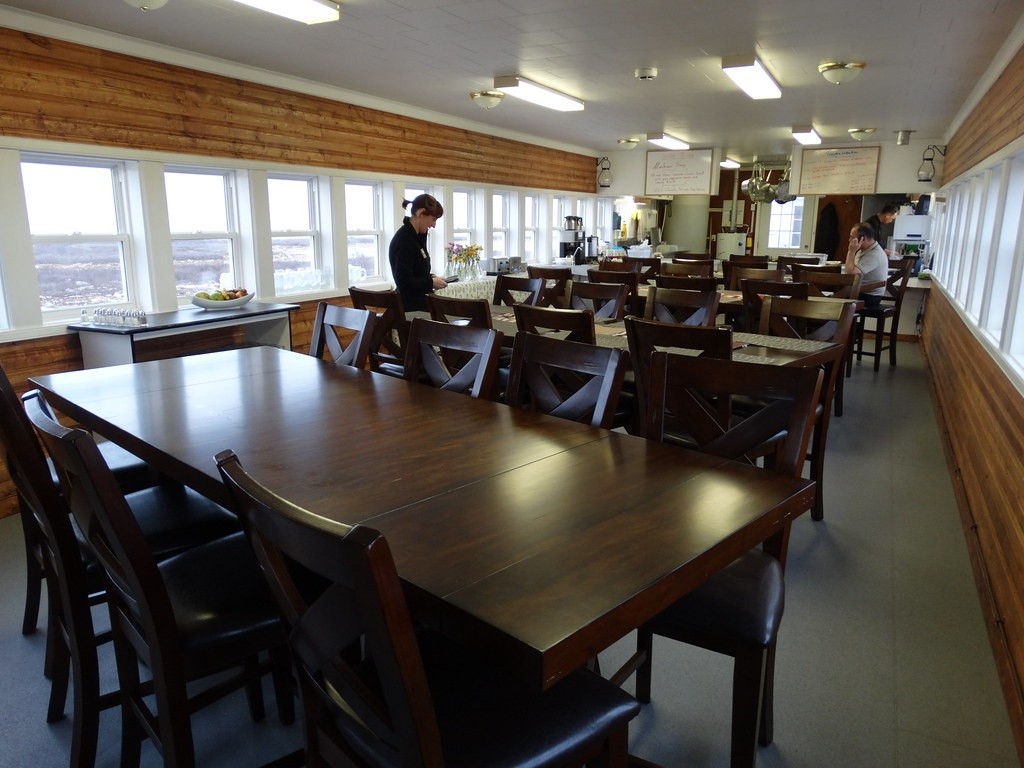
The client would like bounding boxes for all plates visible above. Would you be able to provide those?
[826,261,842,264]
[191,292,256,311]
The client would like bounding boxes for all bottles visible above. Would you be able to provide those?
[81,309,89,324]
[93,307,148,327]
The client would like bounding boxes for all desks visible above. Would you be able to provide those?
[375,304,843,439]
[504,270,865,343]
[26,345,817,768]
[68,301,302,372]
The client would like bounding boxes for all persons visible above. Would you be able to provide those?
[845,222,888,306]
[864,201,902,257]
[389,193,448,312]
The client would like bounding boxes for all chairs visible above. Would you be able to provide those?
[0,253,915,768]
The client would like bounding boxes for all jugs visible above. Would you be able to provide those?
[565,216,583,230]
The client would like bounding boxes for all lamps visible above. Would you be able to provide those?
[918,145,946,181]
[647,132,690,150]
[817,62,865,85]
[792,127,822,145]
[235,0,340,26]
[848,128,877,142]
[617,138,640,149]
[470,93,505,109]
[721,54,783,100]
[597,157,614,188]
[494,75,585,112]
[720,157,740,170]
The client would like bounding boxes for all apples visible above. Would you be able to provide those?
[195,290,225,301]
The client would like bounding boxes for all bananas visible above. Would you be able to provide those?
[221,288,230,300]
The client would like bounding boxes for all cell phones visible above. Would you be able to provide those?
[858,235,864,242]
